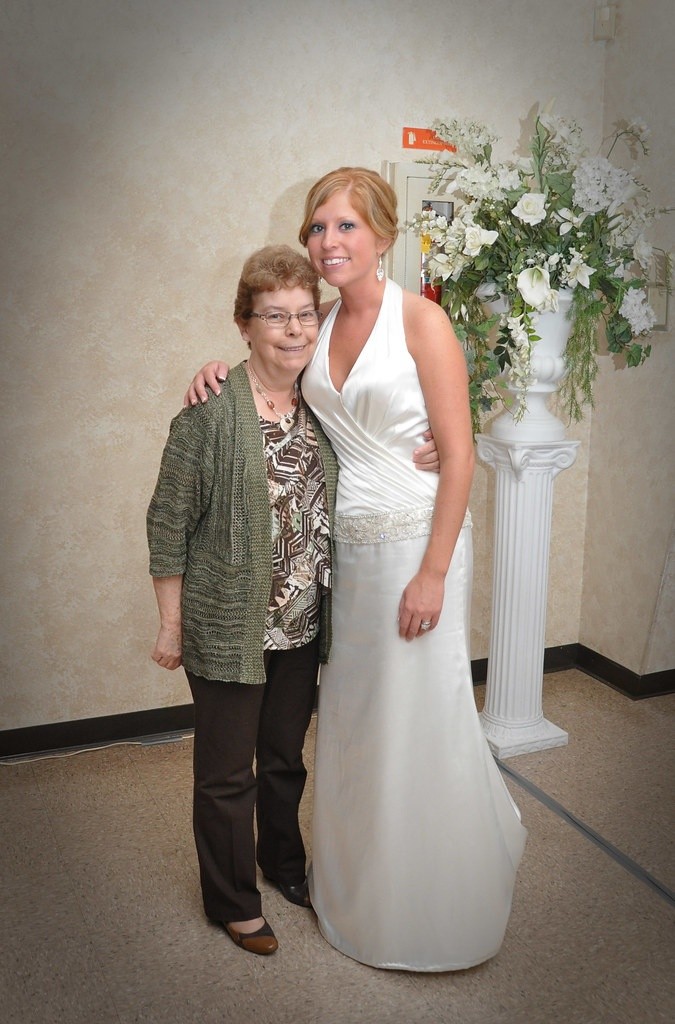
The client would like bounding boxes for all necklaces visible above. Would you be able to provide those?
[247,363,302,433]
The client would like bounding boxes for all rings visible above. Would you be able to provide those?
[422,621,431,631]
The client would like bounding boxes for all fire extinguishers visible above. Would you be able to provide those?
[420,200,442,306]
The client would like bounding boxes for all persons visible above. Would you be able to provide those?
[183,166,529,973]
[145,243,339,954]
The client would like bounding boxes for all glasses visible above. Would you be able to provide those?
[250,310,323,327]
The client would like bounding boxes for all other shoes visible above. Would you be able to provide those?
[221,915,280,954]
[262,869,311,907]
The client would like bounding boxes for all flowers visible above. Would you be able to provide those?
[398,98,674,445]
